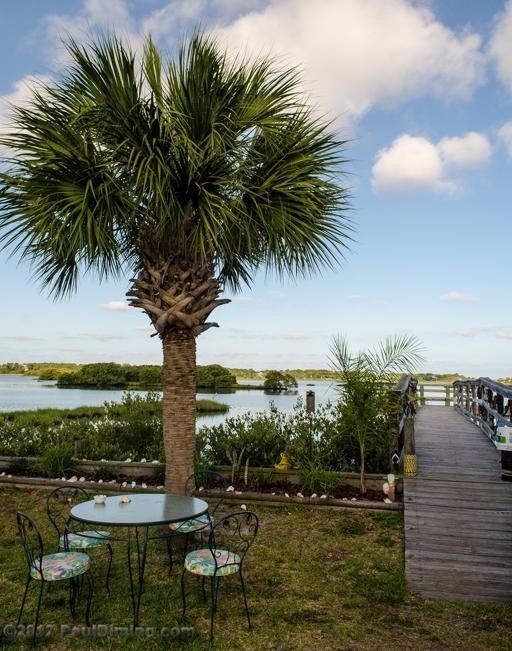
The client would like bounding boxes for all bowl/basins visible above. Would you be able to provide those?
[93,495,107,504]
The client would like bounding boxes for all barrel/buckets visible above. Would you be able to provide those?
[496,427,512,451]
